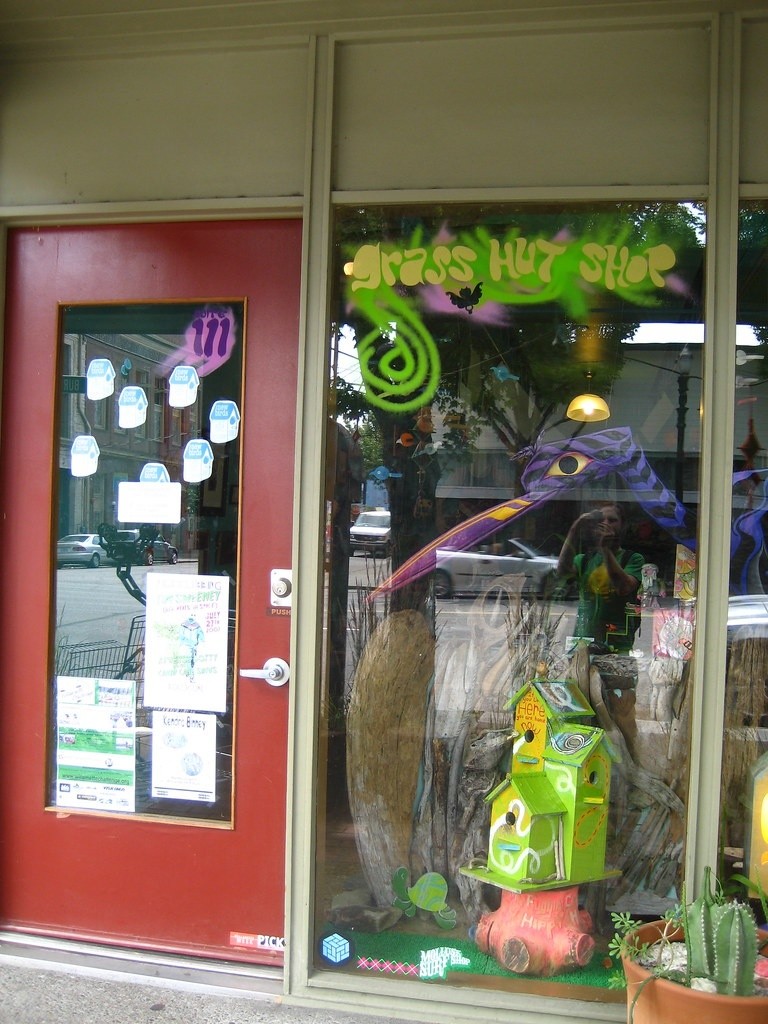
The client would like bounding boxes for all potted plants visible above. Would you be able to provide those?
[607,866,768,1024]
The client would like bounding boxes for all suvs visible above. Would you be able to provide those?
[110,530,178,566]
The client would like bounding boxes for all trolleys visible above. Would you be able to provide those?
[58,614,145,709]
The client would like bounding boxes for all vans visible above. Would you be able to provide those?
[349,511,392,557]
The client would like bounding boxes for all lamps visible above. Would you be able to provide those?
[565,369,610,422]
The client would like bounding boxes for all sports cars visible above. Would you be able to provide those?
[435,537,561,601]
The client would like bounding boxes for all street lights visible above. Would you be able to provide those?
[675,345,693,503]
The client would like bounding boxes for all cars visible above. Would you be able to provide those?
[57,534,117,567]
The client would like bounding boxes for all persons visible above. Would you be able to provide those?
[558,504,645,758]
